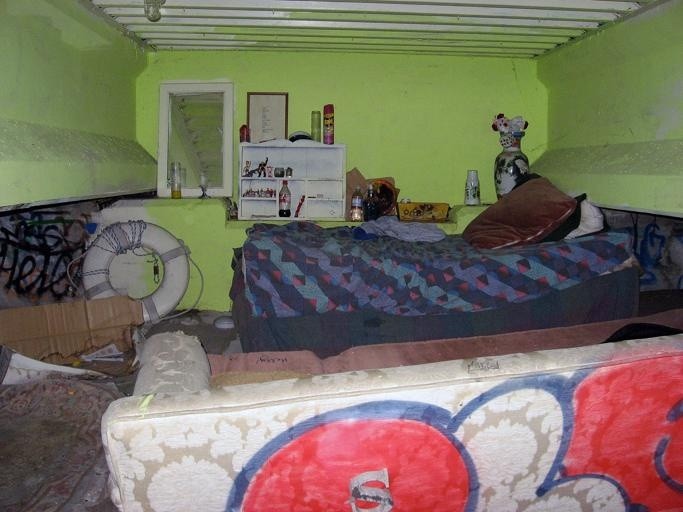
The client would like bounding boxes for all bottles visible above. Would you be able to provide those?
[278,180,292,218]
[239,125,250,145]
[463,167,482,207]
[350,186,363,223]
[362,184,379,222]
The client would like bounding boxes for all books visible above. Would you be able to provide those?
[2,350,112,386]
[80,342,125,364]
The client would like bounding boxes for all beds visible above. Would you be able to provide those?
[231,221,642,351]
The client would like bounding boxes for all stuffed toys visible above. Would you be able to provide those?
[492,113,529,133]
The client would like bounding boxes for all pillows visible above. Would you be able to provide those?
[462,176,581,252]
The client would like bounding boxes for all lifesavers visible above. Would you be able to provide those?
[82,221,188,322]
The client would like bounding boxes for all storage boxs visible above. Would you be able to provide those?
[397,202,451,223]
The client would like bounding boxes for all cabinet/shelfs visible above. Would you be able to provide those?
[239,142,346,221]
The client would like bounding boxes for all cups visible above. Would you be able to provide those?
[170,160,182,199]
[310,109,322,145]
[266,166,293,179]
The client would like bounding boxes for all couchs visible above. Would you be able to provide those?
[100,310,683,512]
[102,198,490,310]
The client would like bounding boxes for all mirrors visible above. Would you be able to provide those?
[367,180,397,215]
[156,82,235,198]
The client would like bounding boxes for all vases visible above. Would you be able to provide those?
[464,170,481,206]
[494,131,529,202]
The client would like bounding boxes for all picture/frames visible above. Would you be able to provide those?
[246,92,288,144]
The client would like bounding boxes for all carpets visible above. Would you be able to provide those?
[0,369,126,512]
